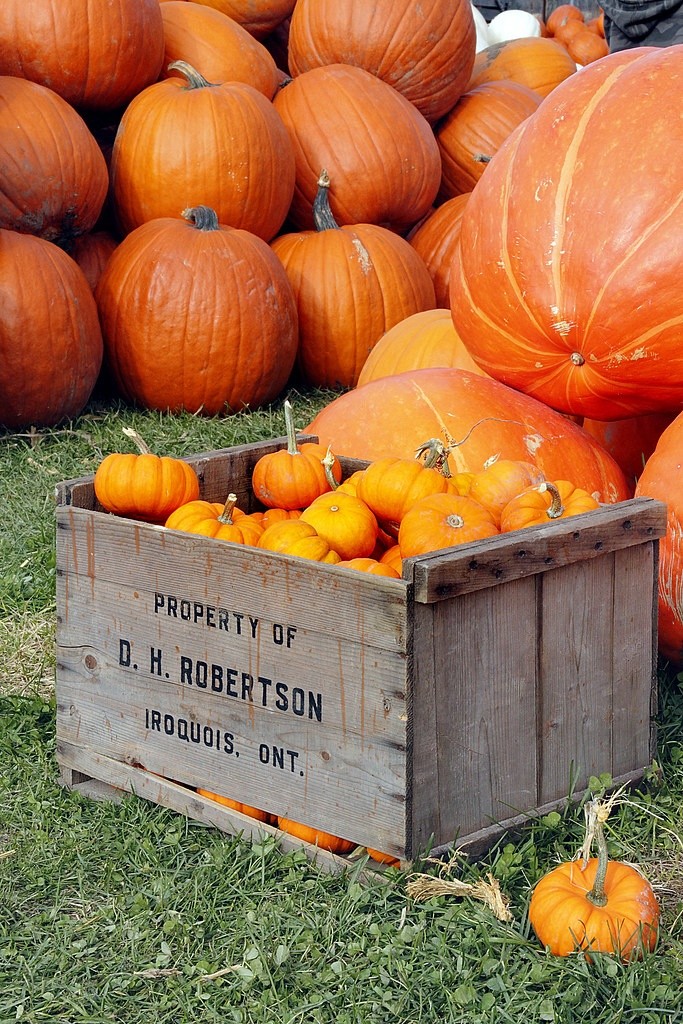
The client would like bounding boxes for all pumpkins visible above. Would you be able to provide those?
[527,809,659,967]
[0,0,683,873]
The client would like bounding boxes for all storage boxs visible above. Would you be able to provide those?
[52,436,667,888]
